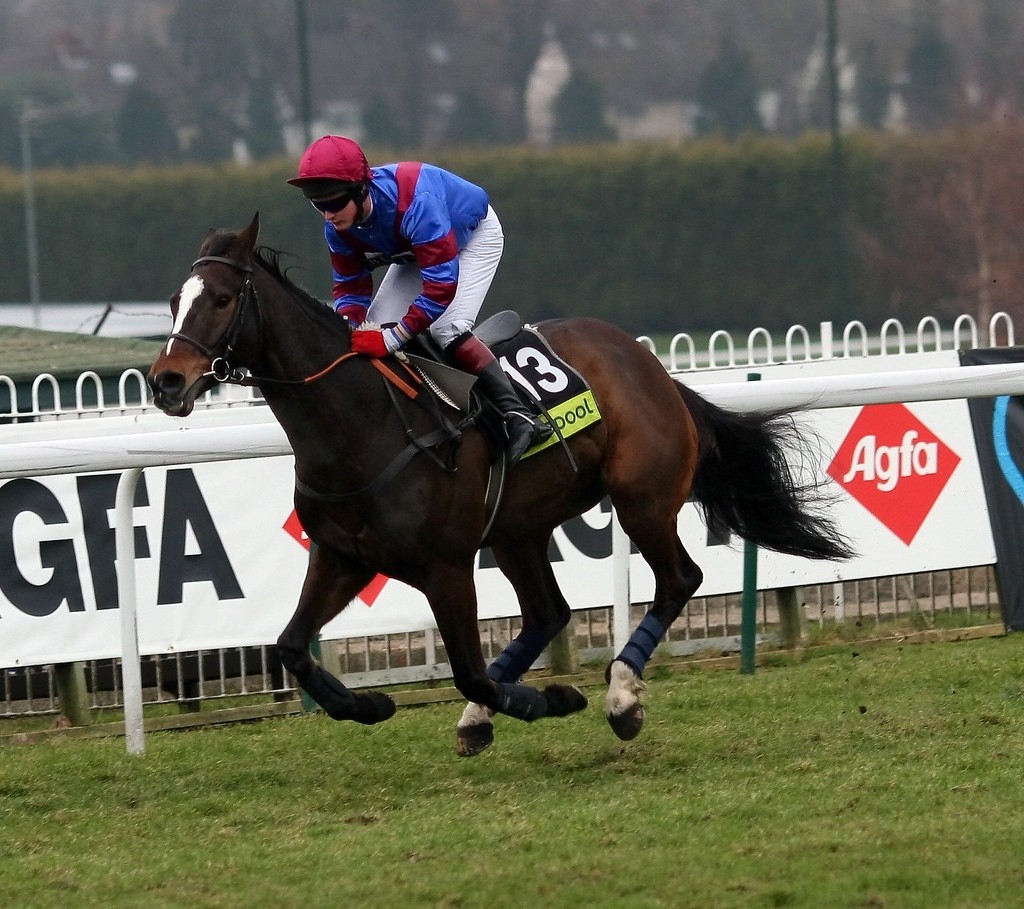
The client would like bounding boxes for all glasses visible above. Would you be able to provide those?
[310,181,364,215]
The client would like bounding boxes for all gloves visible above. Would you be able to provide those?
[351,330,389,359]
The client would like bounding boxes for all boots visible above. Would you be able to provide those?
[448,335,554,470]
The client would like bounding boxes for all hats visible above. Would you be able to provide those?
[286,136,373,188]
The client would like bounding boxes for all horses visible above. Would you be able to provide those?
[144,208,855,758]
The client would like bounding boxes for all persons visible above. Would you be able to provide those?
[288,135,556,456]
